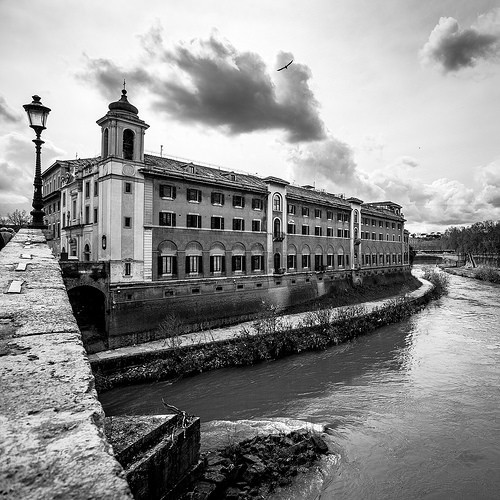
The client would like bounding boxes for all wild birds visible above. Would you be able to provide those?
[277,59,294,72]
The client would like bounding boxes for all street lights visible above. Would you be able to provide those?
[23,94,51,229]
